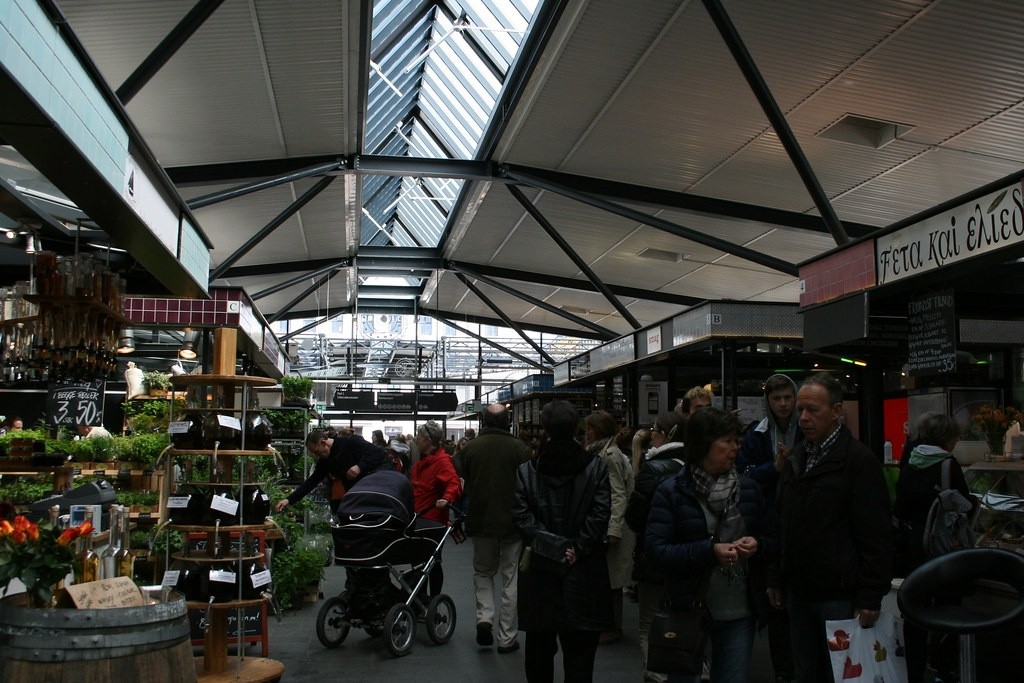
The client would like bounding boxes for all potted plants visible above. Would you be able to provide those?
[0,366,335,614]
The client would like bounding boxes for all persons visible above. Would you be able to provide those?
[735,374,805,683]
[410,420,462,598]
[643,406,778,683]
[275,429,384,613]
[328,429,355,516]
[444,428,475,537]
[510,400,612,683]
[892,411,977,683]
[460,403,532,653]
[765,372,893,683]
[370,430,413,458]
[584,410,636,646]
[681,387,713,418]
[624,412,713,683]
[0,415,23,432]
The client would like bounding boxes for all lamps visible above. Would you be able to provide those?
[179,331,202,359]
[113,328,136,354]
[25,234,34,254]
[6,223,23,239]
[35,239,43,255]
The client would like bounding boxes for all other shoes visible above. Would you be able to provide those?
[498,639,520,652]
[476,619,495,647]
[598,627,625,645]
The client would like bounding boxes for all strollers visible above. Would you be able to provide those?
[316,470,466,658]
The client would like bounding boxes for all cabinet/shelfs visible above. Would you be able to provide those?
[256,406,309,538]
[167,374,285,683]
[123,386,213,439]
[81,460,169,586]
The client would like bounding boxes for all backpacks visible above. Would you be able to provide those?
[923,459,978,558]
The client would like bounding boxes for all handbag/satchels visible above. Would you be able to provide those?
[646,605,710,676]
[826,597,909,683]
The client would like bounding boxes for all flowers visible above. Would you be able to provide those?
[971,403,1024,454]
[0,515,96,606]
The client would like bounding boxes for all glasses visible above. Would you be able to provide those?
[649,427,662,435]
